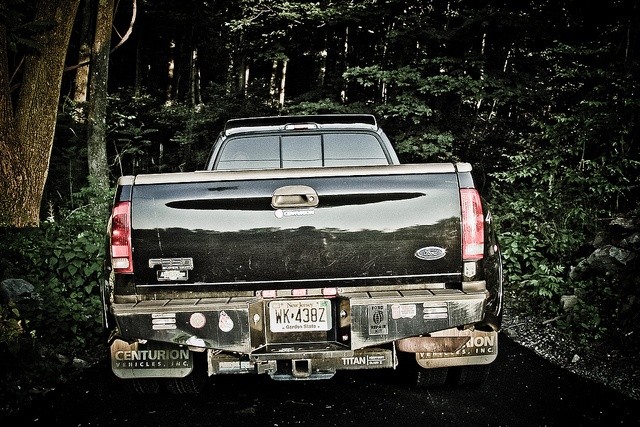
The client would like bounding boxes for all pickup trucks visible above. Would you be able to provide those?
[97,110,512,400]
